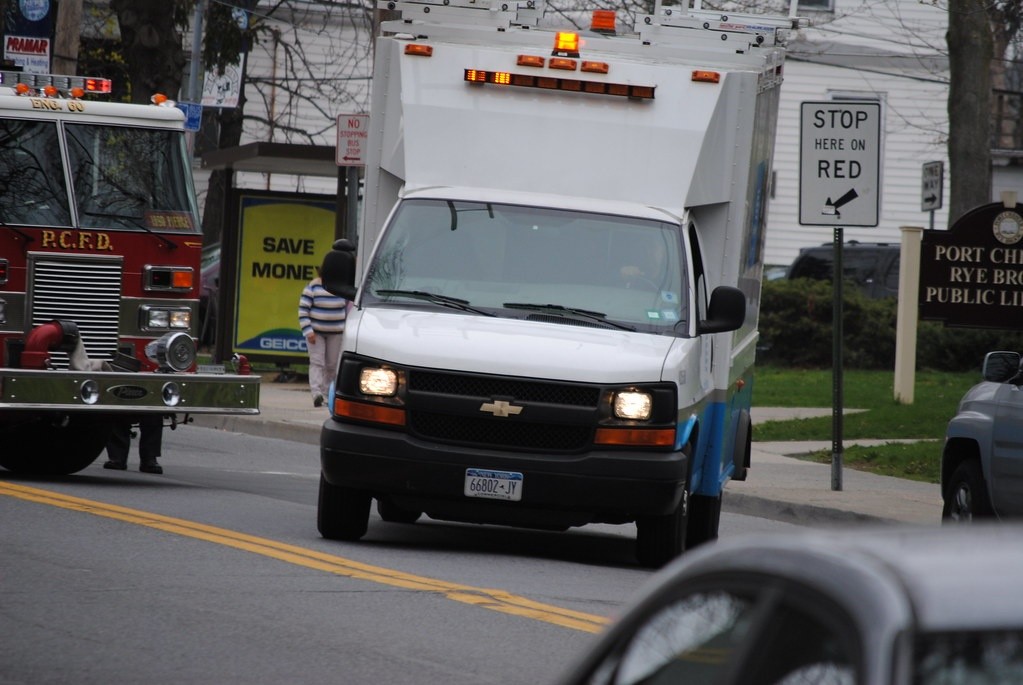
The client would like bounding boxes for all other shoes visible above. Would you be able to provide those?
[104,459,127,469]
[322,394,329,404]
[140,456,162,474]
[313,391,323,407]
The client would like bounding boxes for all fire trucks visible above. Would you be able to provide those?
[0,72,261,477]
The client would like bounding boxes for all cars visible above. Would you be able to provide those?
[942,352,1023,529]
[198,243,224,346]
[548,523,1023,685]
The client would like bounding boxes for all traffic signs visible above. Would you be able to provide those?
[336,114,367,167]
[799,100,880,228]
[922,161,944,212]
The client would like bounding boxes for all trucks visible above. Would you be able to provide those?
[315,0,793,572]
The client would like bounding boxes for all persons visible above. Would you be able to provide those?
[299,277,347,407]
[103,416,164,474]
